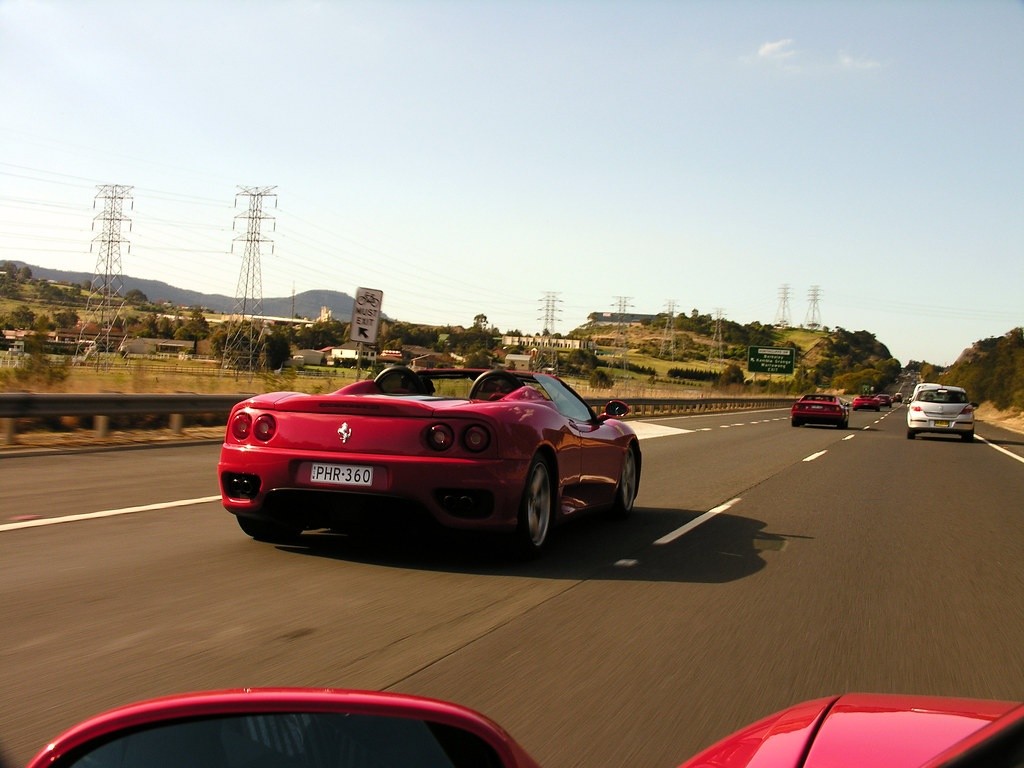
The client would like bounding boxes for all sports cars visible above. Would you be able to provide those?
[217,364,646,560]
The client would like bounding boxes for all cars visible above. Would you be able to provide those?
[905,382,942,404]
[891,393,904,404]
[792,393,850,429]
[904,385,980,443]
[876,395,894,409]
[852,394,881,412]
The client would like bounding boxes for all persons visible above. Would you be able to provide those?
[409,375,525,400]
